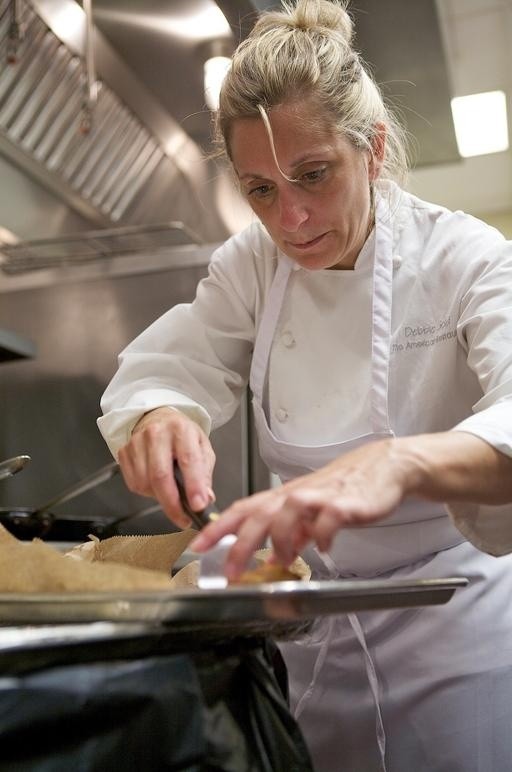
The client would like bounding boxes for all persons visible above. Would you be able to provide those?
[95,0,512,772]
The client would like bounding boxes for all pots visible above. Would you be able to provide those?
[0,450,163,540]
